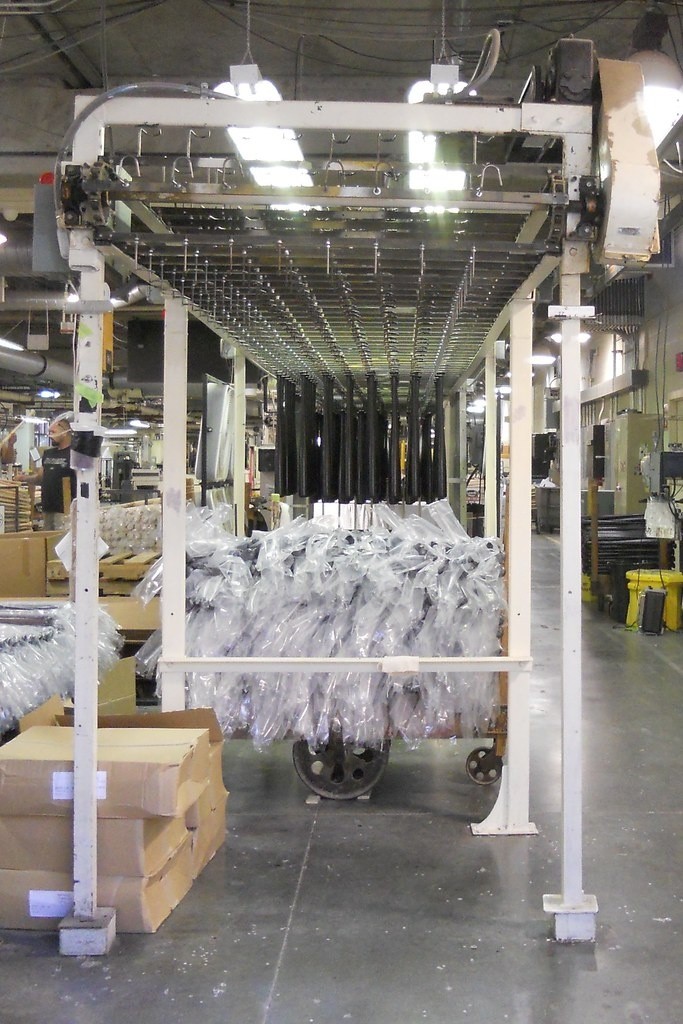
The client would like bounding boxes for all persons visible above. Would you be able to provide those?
[14,419,77,531]
[0,433,17,463]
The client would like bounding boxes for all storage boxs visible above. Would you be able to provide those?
[0,529,72,598]
[0,595,230,934]
